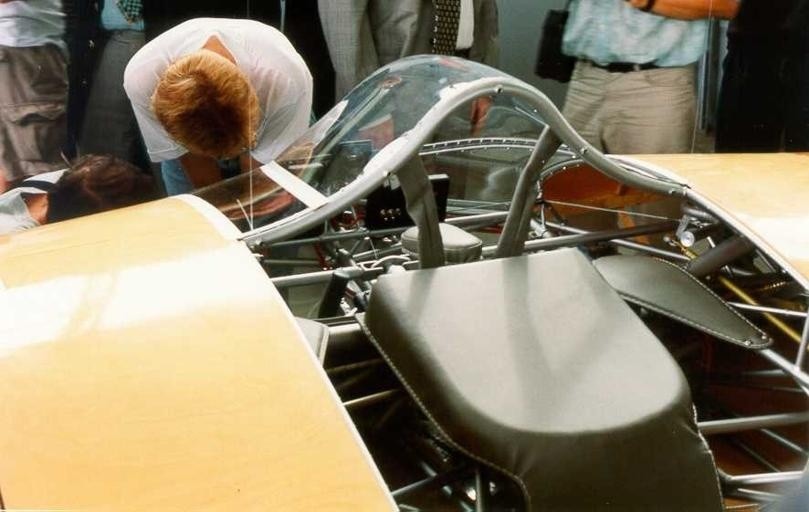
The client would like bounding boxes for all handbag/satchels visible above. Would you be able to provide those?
[536,10,577,82]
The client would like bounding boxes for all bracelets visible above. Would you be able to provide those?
[640,1,657,12]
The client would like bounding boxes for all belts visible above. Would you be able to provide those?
[586,57,656,73]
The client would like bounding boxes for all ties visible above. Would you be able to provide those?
[433,1,461,55]
[117,0,143,22]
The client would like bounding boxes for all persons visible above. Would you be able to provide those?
[715,1,809,153]
[562,1,740,155]
[0,0,501,236]
